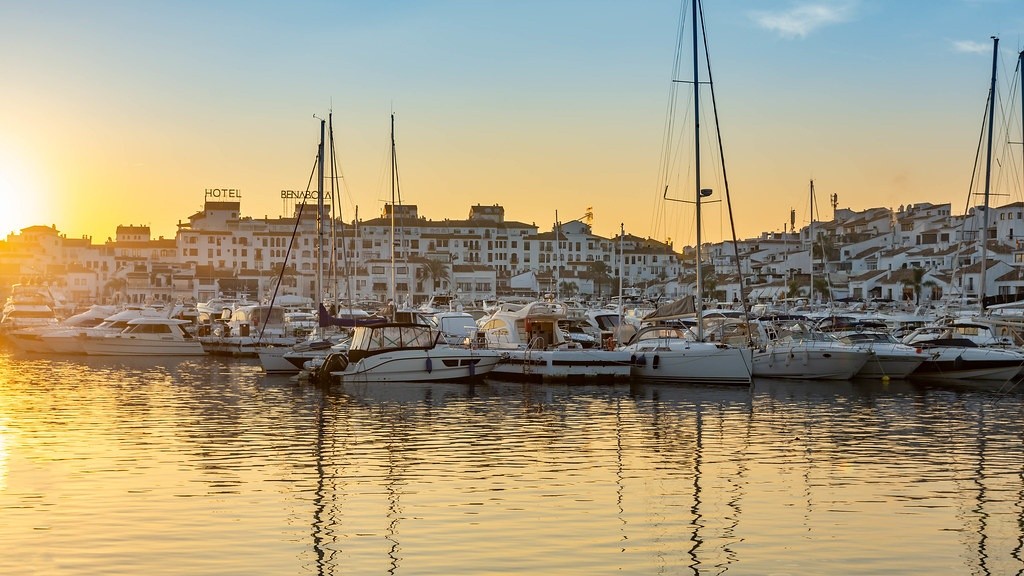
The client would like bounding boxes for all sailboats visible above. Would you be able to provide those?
[304,109,508,381]
[618,0,754,386]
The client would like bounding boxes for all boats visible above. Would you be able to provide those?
[0,36,1019,382]
[73,316,206,355]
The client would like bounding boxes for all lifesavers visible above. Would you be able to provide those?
[855,325,864,333]
[1000,327,1008,336]
